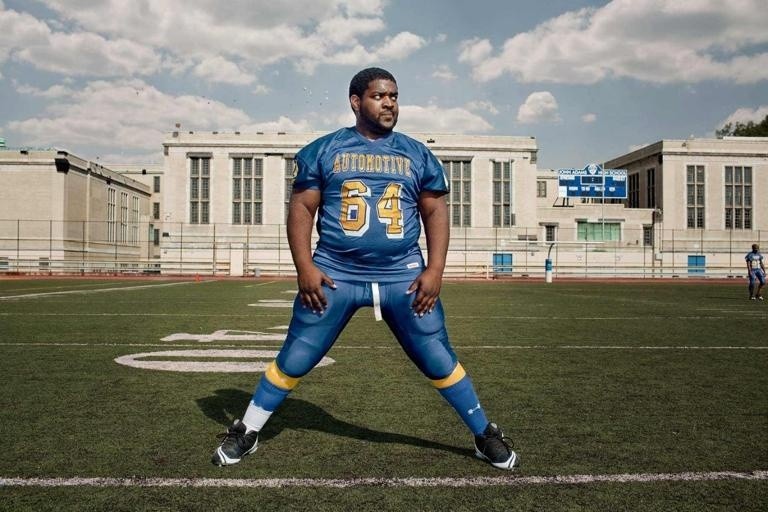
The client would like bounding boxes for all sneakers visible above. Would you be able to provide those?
[212,418,259,465]
[475,422,519,469]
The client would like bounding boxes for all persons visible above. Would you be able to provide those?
[208,66,520,472]
[743,242,767,301]
